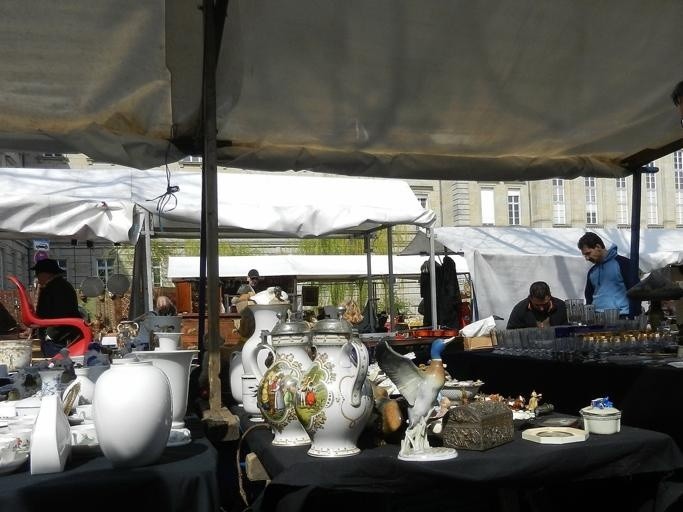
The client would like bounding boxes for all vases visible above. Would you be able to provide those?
[0,332,265,475]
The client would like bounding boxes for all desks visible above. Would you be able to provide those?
[473,341,683,450]
[229,397,682,511]
[181,313,243,348]
[0,424,217,511]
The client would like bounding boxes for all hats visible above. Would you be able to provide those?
[248,270,259,279]
[27,259,66,275]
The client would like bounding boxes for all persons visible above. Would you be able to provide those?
[237,269,260,297]
[576,231,642,319]
[506,281,568,329]
[156,296,177,317]
[27,258,83,358]
[261,374,317,412]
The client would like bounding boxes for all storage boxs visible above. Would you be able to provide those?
[442,401,515,451]
[463,335,493,352]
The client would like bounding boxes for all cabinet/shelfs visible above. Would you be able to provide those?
[172,277,222,313]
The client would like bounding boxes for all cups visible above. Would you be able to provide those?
[241,373,266,423]
[490,297,679,365]
